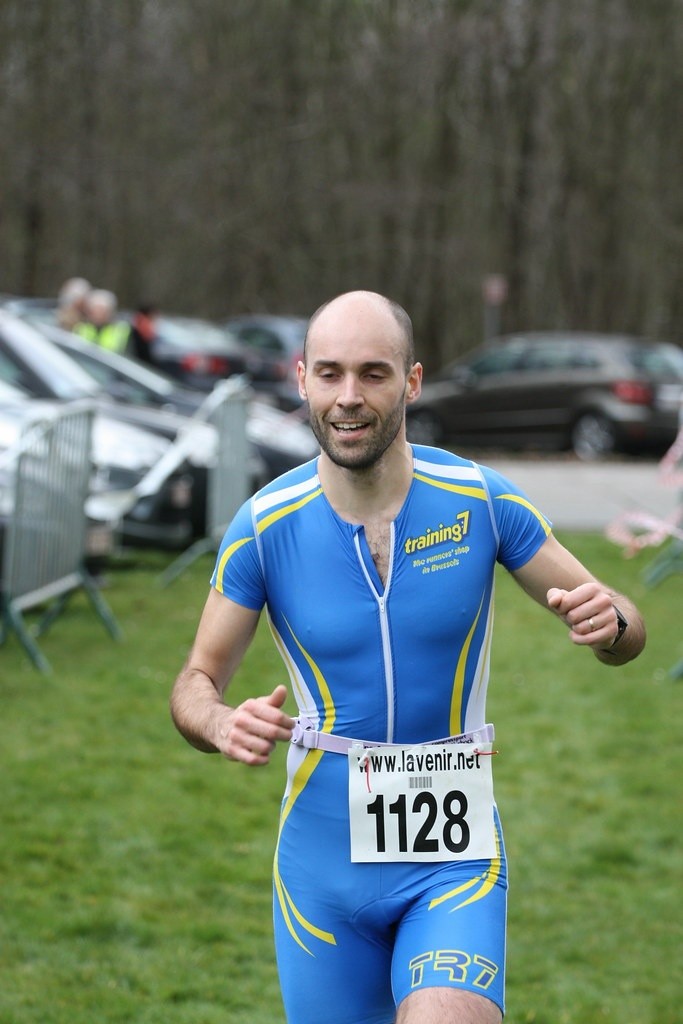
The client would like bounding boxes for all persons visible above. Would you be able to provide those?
[132,304,197,383]
[172,289,648,1023]
[56,278,99,344]
[80,291,133,357]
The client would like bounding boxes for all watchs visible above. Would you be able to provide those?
[600,604,628,652]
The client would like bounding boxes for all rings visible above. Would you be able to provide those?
[588,619,595,631]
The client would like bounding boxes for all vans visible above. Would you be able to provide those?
[404,329,683,466]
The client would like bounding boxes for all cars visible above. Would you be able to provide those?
[0,294,315,552]
